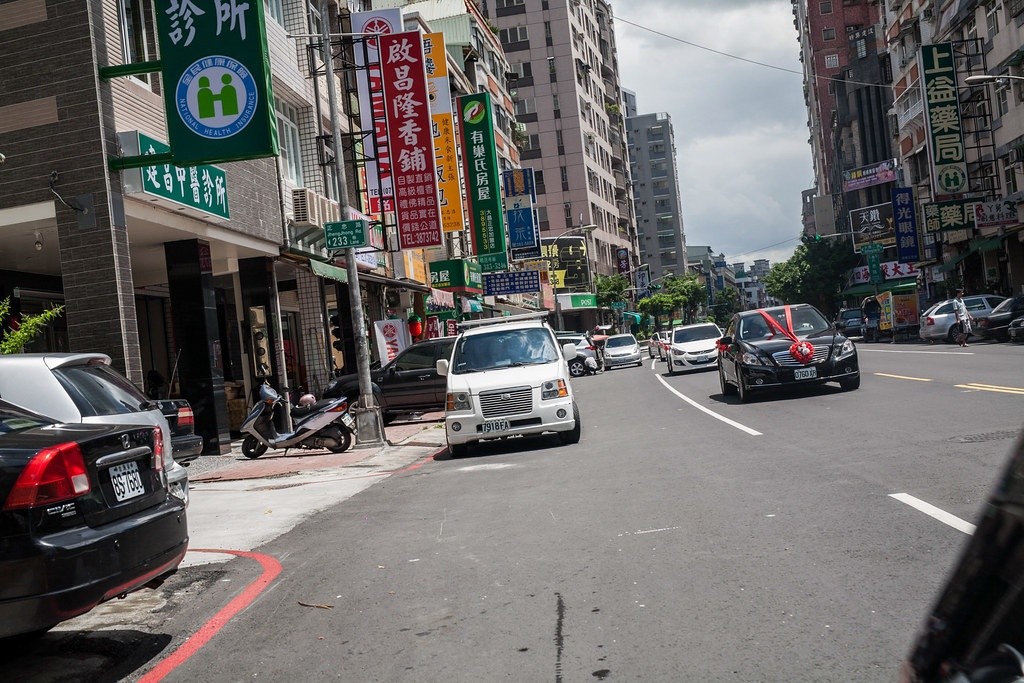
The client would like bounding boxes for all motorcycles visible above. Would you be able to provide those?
[858,282,920,344]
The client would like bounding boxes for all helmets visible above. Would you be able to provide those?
[299,394,316,407]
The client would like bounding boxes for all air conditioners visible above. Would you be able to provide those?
[292,187,339,227]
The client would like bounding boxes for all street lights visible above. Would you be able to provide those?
[550,224,598,331]
[704,303,728,321]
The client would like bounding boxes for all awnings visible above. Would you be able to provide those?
[978,223,1024,254]
[622,311,641,324]
[637,312,655,326]
[939,234,995,274]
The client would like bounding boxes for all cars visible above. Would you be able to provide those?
[1007,314,1024,344]
[602,333,643,371]
[648,322,728,376]
[832,306,867,338]
[918,293,1009,345]
[715,302,861,403]
[971,293,1024,343]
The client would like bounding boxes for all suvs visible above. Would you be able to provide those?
[322,334,460,428]
[0,351,206,669]
[525,330,602,377]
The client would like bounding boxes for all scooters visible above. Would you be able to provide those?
[240,364,358,459]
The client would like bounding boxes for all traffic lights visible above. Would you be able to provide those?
[801,233,821,243]
[647,284,661,289]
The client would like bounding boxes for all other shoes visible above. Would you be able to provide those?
[956,338,961,346]
[963,343,969,347]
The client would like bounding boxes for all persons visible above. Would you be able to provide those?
[952,289,973,347]
[146,369,169,400]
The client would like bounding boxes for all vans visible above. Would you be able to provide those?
[435,309,581,459]
